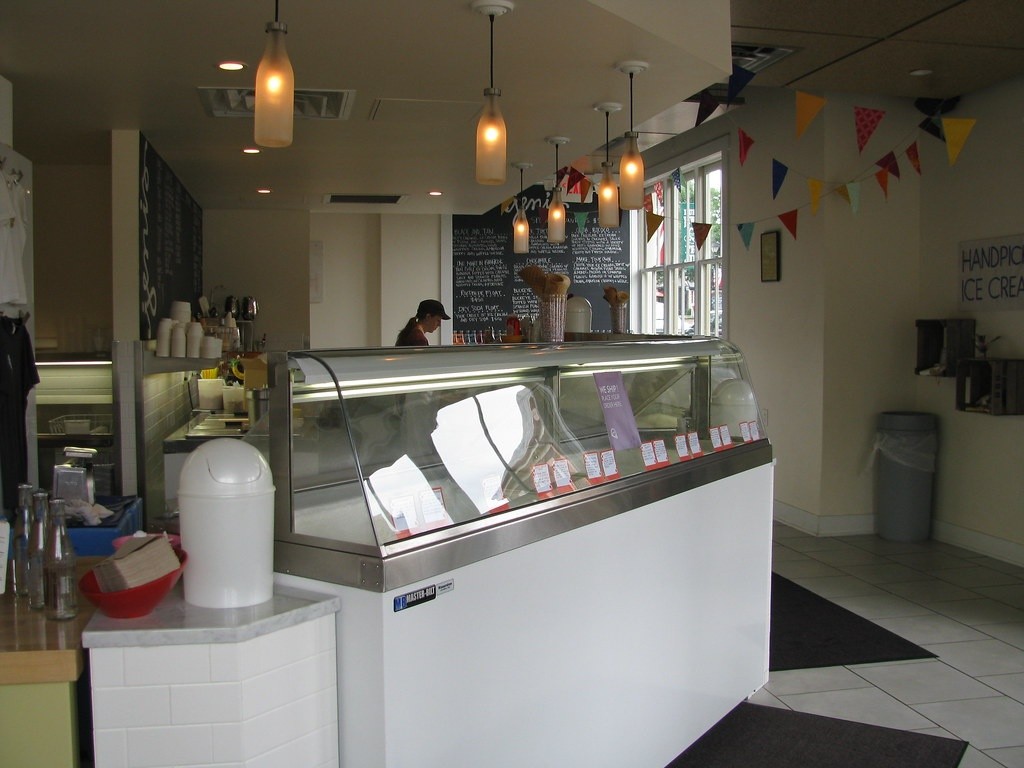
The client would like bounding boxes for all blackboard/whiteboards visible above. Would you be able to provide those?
[452,185,630,334]
[139,131,204,341]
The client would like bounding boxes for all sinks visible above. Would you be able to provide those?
[185,414,246,439]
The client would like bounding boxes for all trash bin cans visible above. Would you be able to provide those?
[567,296,592,333]
[875,410,938,541]
[710,378,758,437]
[177,439,276,609]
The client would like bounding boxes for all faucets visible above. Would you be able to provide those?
[188,410,216,433]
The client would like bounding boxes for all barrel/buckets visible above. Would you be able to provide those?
[225,296,238,319]
[197,379,244,409]
[242,297,258,321]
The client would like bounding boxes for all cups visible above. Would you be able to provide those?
[155,301,223,358]
[484,330,494,343]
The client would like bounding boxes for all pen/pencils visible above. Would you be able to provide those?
[262,333,266,342]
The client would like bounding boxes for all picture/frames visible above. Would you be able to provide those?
[760,230,781,282]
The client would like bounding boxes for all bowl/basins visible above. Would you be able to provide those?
[78,547,188,618]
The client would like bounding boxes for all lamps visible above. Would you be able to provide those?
[544,135,572,242]
[253,0,295,148]
[614,59,649,209]
[511,161,534,254]
[593,101,623,228]
[469,0,515,183]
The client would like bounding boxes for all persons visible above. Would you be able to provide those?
[394,298,450,414]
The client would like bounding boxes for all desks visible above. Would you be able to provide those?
[83,583,338,768]
[0,593,94,768]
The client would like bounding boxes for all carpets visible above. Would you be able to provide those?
[668,701,970,768]
[769,569,940,671]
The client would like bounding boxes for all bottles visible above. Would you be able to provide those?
[217,312,240,351]
[453,330,483,344]
[498,330,501,342]
[15,484,33,596]
[41,499,75,619]
[29,493,50,607]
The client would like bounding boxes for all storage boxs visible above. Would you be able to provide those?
[221,385,246,414]
[197,378,224,411]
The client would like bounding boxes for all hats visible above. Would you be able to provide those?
[417,300,451,320]
